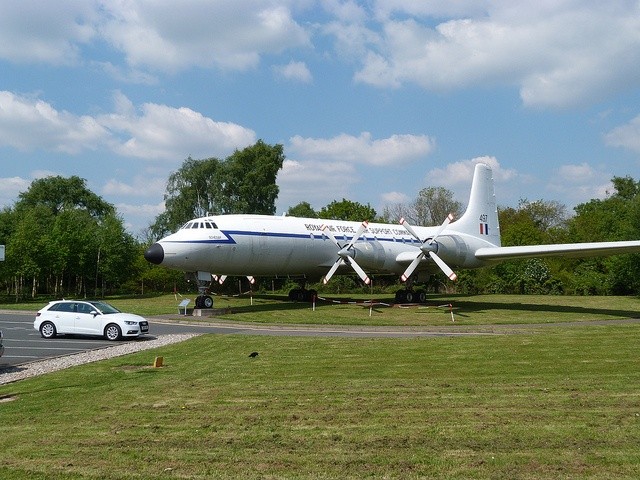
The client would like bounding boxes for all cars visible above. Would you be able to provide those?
[33,298,149,341]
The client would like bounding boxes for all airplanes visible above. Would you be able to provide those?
[145,162,639,309]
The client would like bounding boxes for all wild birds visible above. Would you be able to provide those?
[248,351,259,359]
